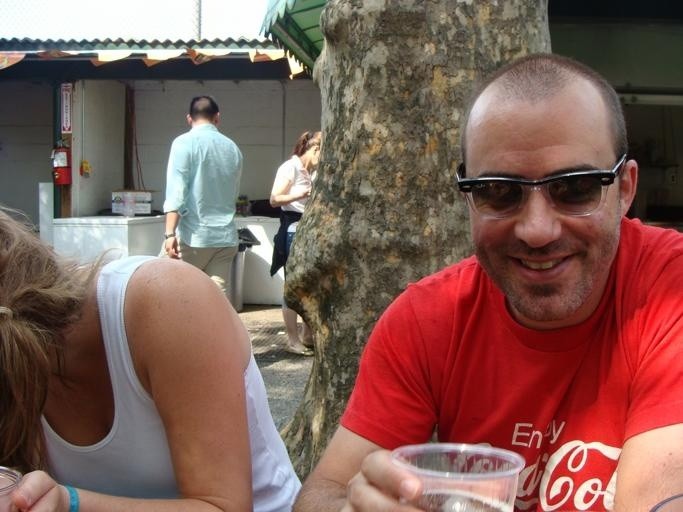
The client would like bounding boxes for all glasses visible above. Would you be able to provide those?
[453,152,627,220]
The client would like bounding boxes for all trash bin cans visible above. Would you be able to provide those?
[227,241,247,312]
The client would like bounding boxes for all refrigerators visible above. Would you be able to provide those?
[240,216,286,305]
[51,214,246,317]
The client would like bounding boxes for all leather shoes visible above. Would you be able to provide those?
[285,338,316,356]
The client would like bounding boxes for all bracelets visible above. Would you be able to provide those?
[649,494,681,512]
[163,232,176,240]
[62,484,80,512]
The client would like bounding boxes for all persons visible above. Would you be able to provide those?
[1,201,305,512]
[158,92,245,304]
[290,51,682,512]
[267,130,322,357]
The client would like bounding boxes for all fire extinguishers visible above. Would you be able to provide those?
[50,137,71,185]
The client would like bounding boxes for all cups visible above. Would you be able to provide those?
[123,193,135,219]
[393,444,526,512]
